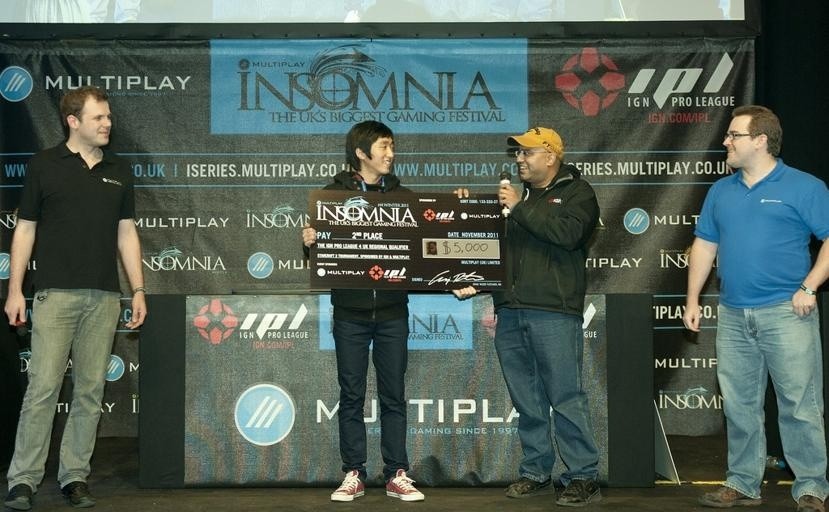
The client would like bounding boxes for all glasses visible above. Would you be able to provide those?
[724,132,753,141]
[514,150,548,157]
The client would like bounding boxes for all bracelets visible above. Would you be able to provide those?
[800,283,817,296]
[131,287,146,295]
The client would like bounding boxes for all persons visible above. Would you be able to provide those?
[453,125,609,505]
[3,86,149,511]
[299,121,477,508]
[683,104,829,510]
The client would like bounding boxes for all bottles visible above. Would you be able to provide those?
[766,455,786,470]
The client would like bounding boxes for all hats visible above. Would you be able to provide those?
[507,127,564,160]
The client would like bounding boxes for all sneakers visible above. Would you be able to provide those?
[503,476,555,498]
[556,480,602,506]
[700,486,762,507]
[62,481,96,508]
[331,469,364,502]
[797,495,825,512]
[386,468,424,501]
[4,484,32,510]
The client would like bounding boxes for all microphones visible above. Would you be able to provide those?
[499,170,511,220]
